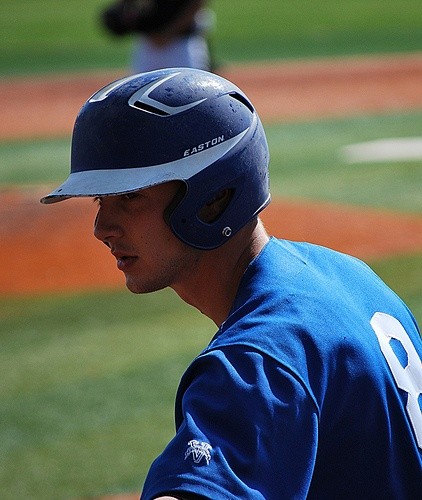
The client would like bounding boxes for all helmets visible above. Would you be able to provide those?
[39,66,272,250]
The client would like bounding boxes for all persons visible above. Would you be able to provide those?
[39,65,422,500]
[101,0,219,78]
